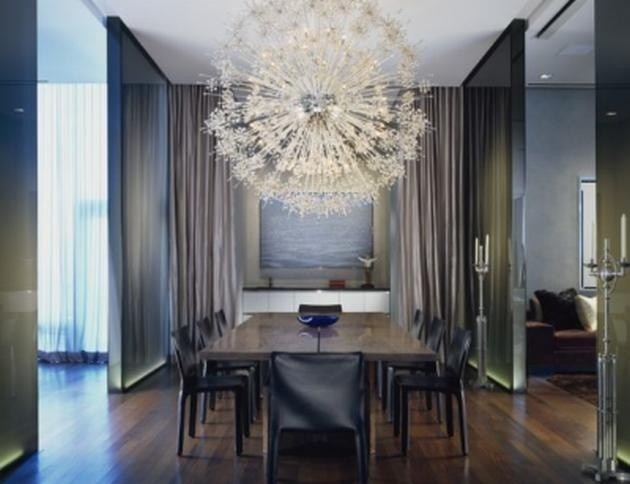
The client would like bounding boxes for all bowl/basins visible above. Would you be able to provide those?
[298,305,342,327]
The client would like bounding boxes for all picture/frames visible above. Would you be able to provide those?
[258,190,376,270]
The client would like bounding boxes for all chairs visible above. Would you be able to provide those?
[526,307,596,379]
[213,308,265,402]
[379,304,424,406]
[263,350,371,484]
[380,315,451,425]
[168,324,253,456]
[194,316,260,427]
[394,326,476,457]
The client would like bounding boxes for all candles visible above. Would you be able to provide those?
[620,212,627,258]
[604,237,610,249]
[473,233,491,266]
[589,220,595,260]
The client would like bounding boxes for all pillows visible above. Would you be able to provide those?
[530,286,599,334]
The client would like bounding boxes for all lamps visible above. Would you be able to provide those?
[196,1,439,220]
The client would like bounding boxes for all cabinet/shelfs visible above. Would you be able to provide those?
[240,289,391,316]
